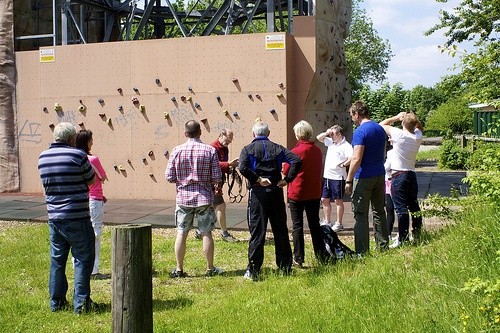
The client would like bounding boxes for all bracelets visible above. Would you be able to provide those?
[342,163,345,168]
[345,180,353,184]
[256,177,262,182]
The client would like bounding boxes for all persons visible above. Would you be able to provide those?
[37,121,107,316]
[280,120,335,267]
[238,121,304,282]
[72,130,112,280]
[192,129,241,244]
[344,101,389,260]
[165,120,222,278]
[382,132,401,240]
[315,125,355,232]
[377,110,424,249]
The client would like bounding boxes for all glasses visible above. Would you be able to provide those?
[224,135,234,141]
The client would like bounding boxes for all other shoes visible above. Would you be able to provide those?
[92,273,107,279]
[168,269,184,278]
[78,299,106,314]
[409,235,418,244]
[330,221,344,233]
[276,268,290,276]
[52,301,74,312]
[221,233,240,243]
[388,239,402,249]
[392,233,399,240]
[244,269,259,281]
[320,220,333,228]
[205,266,223,277]
[292,256,303,269]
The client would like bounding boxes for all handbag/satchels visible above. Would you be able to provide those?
[102,196,107,203]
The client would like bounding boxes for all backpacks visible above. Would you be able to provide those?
[320,224,356,265]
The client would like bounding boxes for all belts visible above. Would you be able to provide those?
[388,170,408,180]
